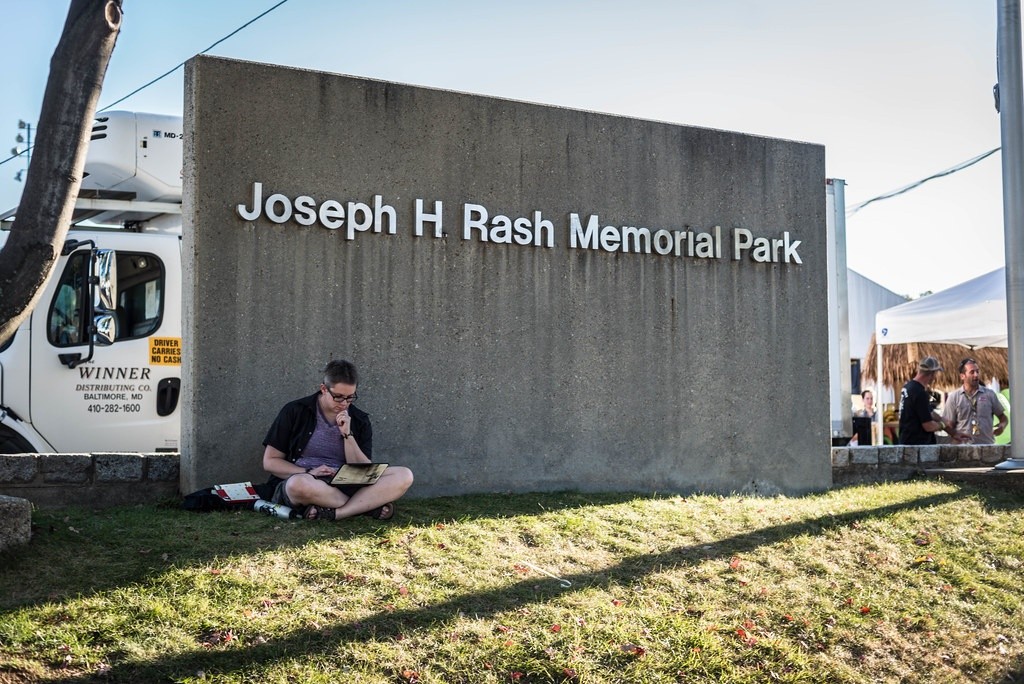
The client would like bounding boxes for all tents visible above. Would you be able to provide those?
[874,265,1010,445]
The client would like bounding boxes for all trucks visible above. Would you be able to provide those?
[0,111,182,457]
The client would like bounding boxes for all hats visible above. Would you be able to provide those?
[920,357,944,371]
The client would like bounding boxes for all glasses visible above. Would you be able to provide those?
[323,382,358,403]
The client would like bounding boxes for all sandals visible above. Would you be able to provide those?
[303,504,336,522]
[364,503,394,519]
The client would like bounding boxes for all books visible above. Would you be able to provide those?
[210,481,260,501]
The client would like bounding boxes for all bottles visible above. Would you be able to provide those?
[254,500,304,521]
[971,412,981,436]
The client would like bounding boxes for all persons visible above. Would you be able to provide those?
[852,391,879,423]
[890,356,1011,446]
[257,358,414,521]
